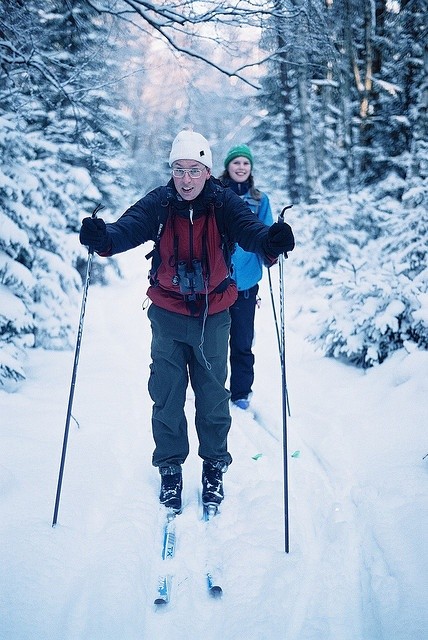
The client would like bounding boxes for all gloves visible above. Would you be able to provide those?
[80,217,112,253]
[264,254,278,267]
[265,222,295,259]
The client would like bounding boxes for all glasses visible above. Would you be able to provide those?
[170,167,206,178]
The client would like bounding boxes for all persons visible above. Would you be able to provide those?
[219,145,275,409]
[80,129,294,507]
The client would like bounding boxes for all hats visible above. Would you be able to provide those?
[224,144,253,171]
[169,131,212,174]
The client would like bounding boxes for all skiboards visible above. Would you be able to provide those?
[251,450,300,461]
[154,504,223,604]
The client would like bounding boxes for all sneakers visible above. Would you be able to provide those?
[159,466,182,509]
[201,459,225,506]
